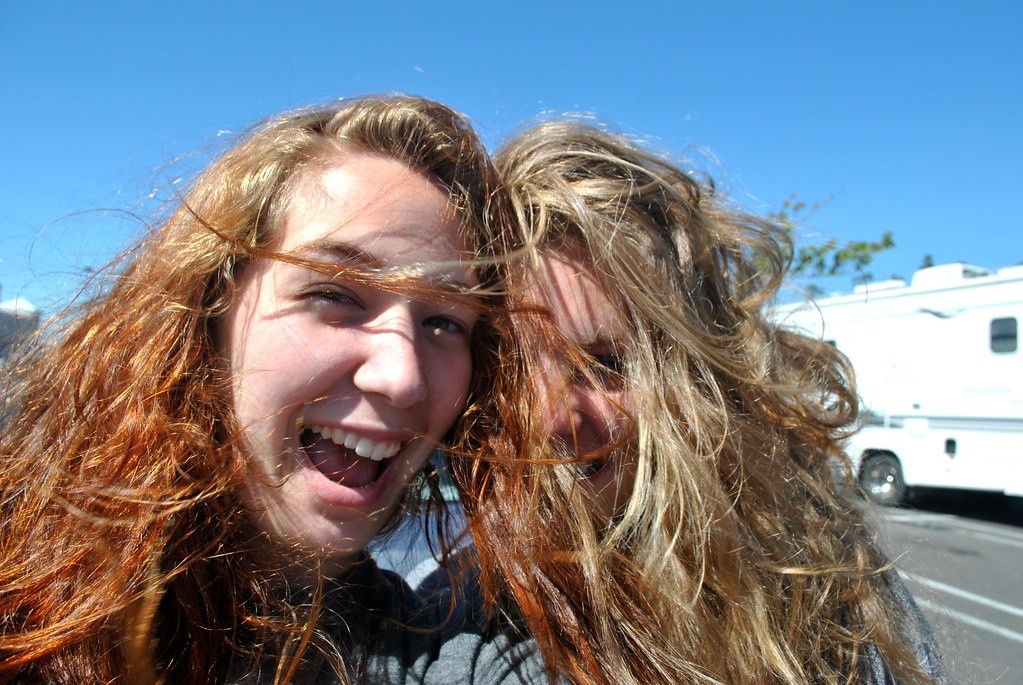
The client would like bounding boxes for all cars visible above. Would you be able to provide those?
[361,480,483,598]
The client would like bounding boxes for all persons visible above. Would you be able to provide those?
[399,109,950,683]
[0,88,495,685]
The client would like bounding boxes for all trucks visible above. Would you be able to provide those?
[749,260,1023,516]
